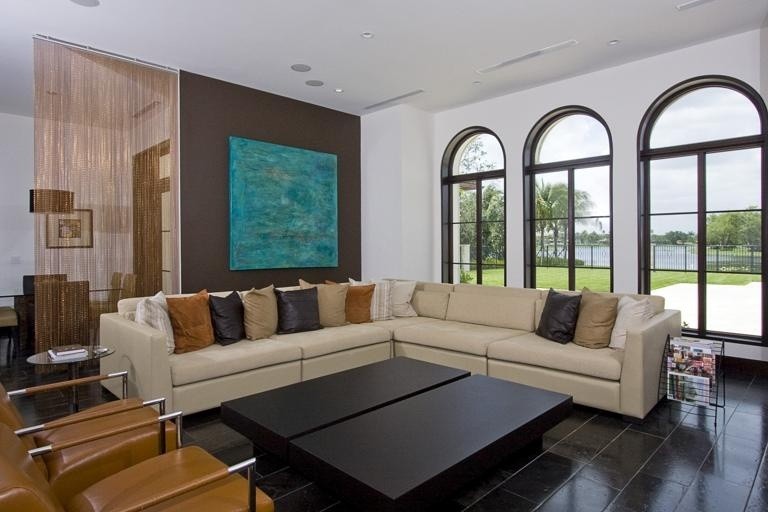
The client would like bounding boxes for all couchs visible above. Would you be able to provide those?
[100,290,680,423]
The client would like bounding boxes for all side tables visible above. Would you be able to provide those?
[26,346,115,414]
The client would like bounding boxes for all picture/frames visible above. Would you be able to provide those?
[45,209,94,249]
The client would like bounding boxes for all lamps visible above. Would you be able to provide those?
[29,91,73,213]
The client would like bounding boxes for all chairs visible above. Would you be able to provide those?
[0,370,177,478]
[0,272,139,356]
[0,411,274,511]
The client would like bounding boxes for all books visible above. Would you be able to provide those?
[667,344,715,406]
[47,343,89,362]
